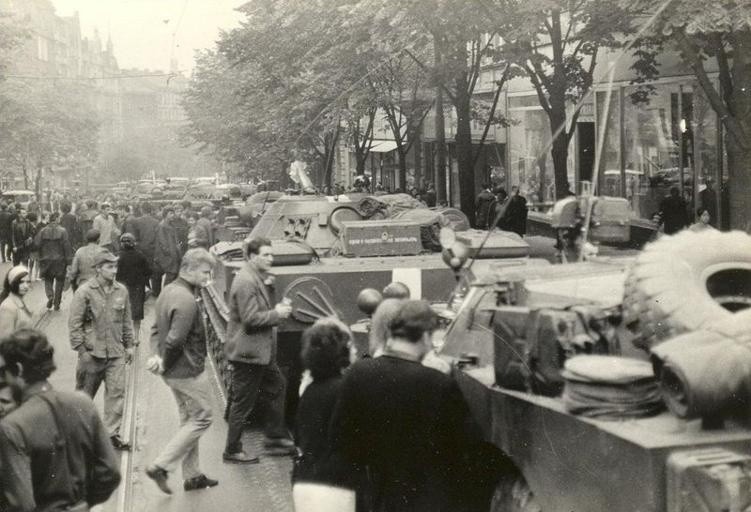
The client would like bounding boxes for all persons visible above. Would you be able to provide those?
[658,187,687,234]
[144,248,219,495]
[323,180,383,194]
[0,327,121,510]
[285,316,361,511]
[68,252,136,450]
[1,264,34,365]
[223,238,294,464]
[690,207,714,232]
[475,182,527,237]
[326,300,484,510]
[396,182,437,208]
[1,194,214,349]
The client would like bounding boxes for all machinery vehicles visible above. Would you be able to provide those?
[204,188,285,243]
[196,158,552,409]
[339,178,751,512]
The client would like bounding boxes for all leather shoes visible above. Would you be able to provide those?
[111,435,132,449]
[146,464,173,495]
[184,474,219,491]
[223,451,259,465]
[262,438,294,449]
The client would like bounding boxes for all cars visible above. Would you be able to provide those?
[0,176,253,212]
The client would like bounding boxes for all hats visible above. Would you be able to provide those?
[89,250,120,268]
